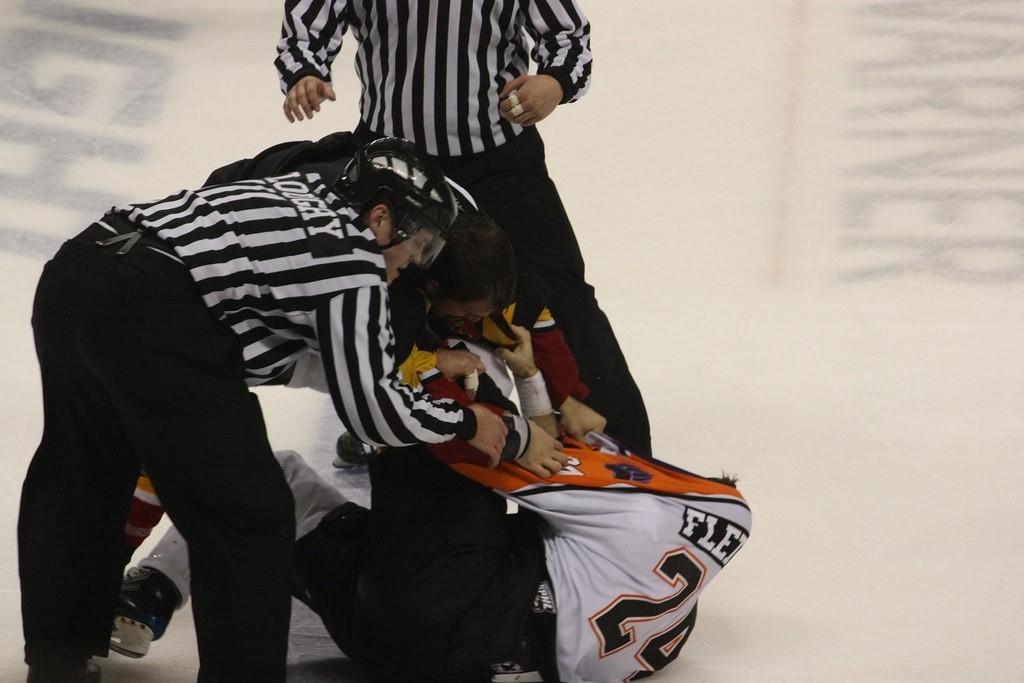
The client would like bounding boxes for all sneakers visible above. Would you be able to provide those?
[109,567,182,659]
[332,430,384,472]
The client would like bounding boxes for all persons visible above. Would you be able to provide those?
[109,323,753,683]
[274,0,655,465]
[16,136,510,680]
[203,129,608,480]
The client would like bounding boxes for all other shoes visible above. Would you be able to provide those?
[26,659,101,683]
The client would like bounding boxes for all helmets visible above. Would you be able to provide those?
[331,137,483,270]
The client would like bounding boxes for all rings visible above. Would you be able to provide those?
[510,104,524,117]
[509,94,520,107]
